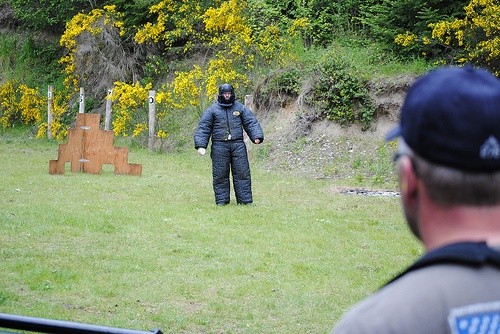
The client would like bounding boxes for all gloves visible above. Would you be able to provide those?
[198,148,206,156]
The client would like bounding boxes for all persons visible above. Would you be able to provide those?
[192,83,263,205]
[331,63,499,334]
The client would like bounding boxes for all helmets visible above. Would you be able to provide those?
[216,84,237,107]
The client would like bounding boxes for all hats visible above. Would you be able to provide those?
[386,64,500,174]
